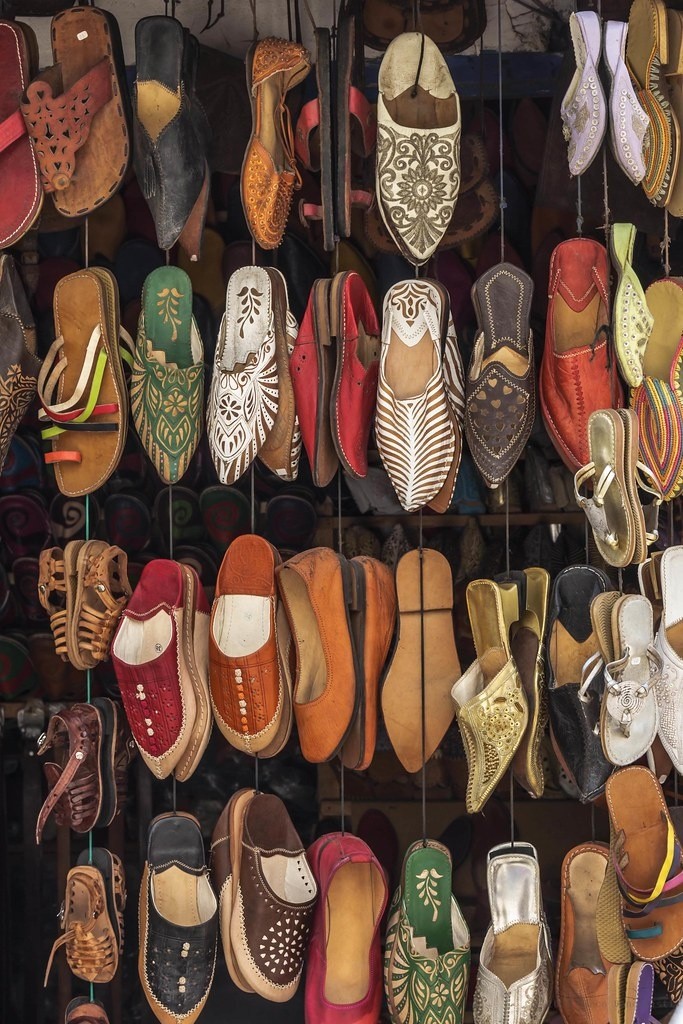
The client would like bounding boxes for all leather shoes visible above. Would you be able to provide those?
[0,264,683,1024]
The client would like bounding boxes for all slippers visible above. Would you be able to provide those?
[560,11,649,187]
[0,6,211,263]
[625,1,683,220]
[296,15,376,252]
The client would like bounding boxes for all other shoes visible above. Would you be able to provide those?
[376,32,462,267]
[240,37,312,250]
